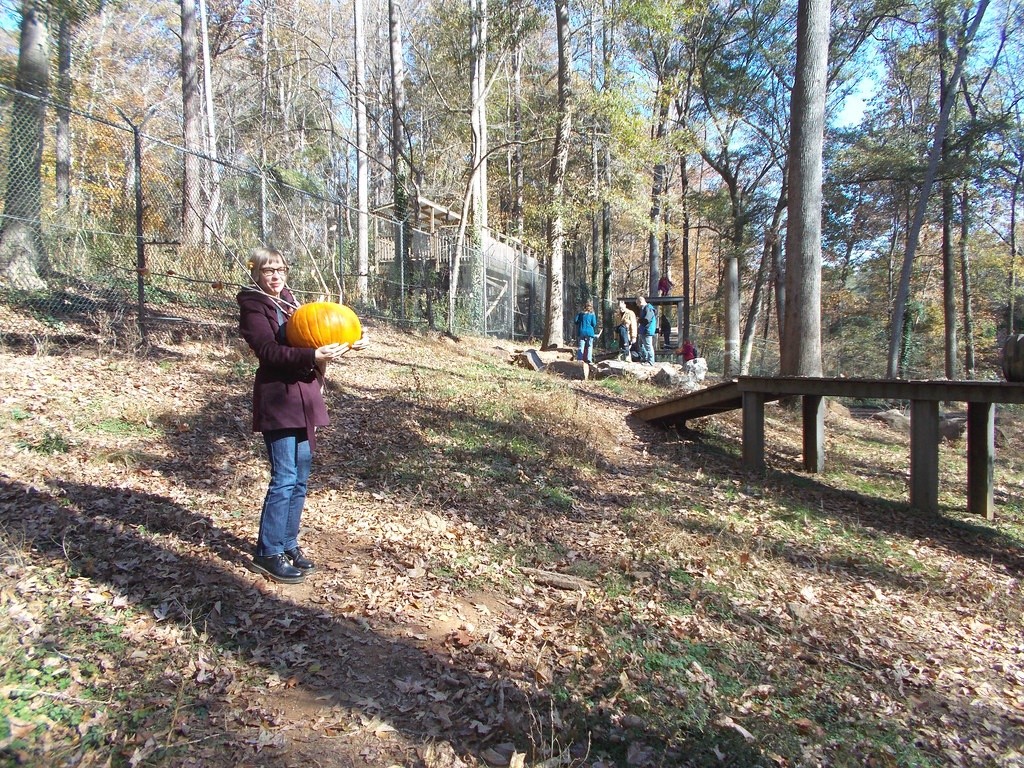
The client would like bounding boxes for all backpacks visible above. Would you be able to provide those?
[687,344,697,358]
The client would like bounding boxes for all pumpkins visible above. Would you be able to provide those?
[285,295,361,350]
[676,348,683,355]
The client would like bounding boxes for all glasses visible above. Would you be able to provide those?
[259,267,290,276]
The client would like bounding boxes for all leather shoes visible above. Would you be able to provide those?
[284,547,315,572]
[250,552,306,583]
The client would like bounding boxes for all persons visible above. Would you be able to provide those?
[659,314,671,345]
[574,301,596,364]
[235,248,372,583]
[611,300,637,362]
[677,339,695,363]
[658,272,674,297]
[634,296,656,366]
